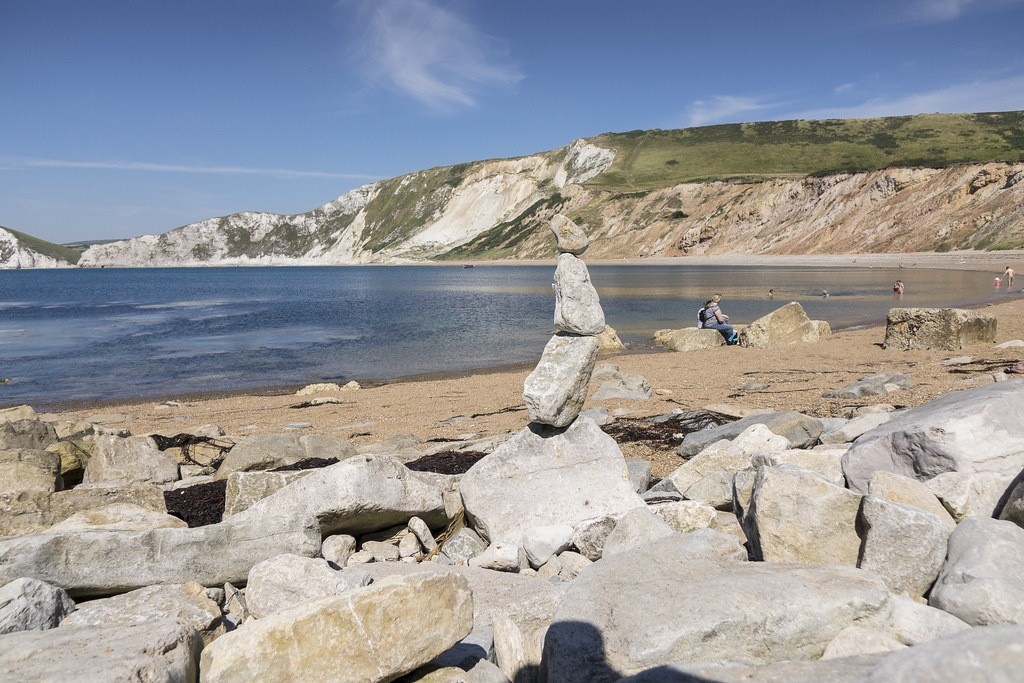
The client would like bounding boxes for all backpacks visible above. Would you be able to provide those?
[699,306,715,322]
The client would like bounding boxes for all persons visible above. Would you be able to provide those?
[894,280,904,294]
[769,289,774,295]
[696,294,737,344]
[823,290,829,296]
[994,266,1015,286]
